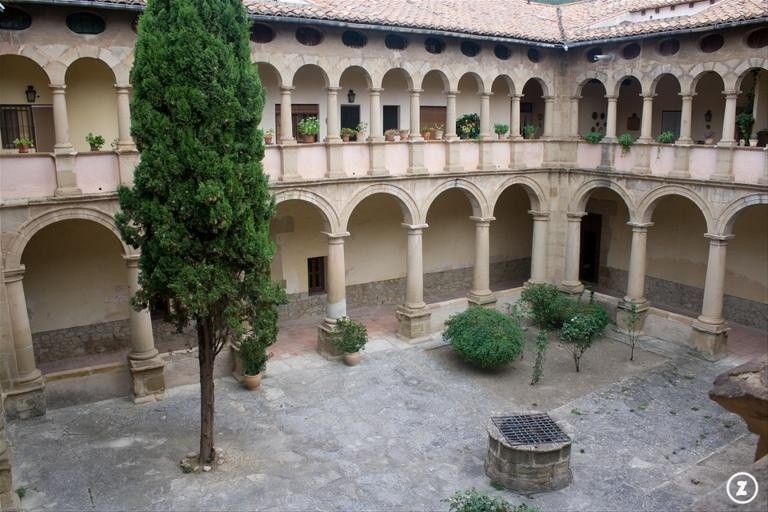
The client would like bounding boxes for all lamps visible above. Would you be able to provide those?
[25,85,36,102]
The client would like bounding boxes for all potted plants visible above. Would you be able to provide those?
[236,335,269,390]
[13,137,34,153]
[748,130,759,147]
[263,116,536,144]
[329,315,369,366]
[85,131,105,151]
